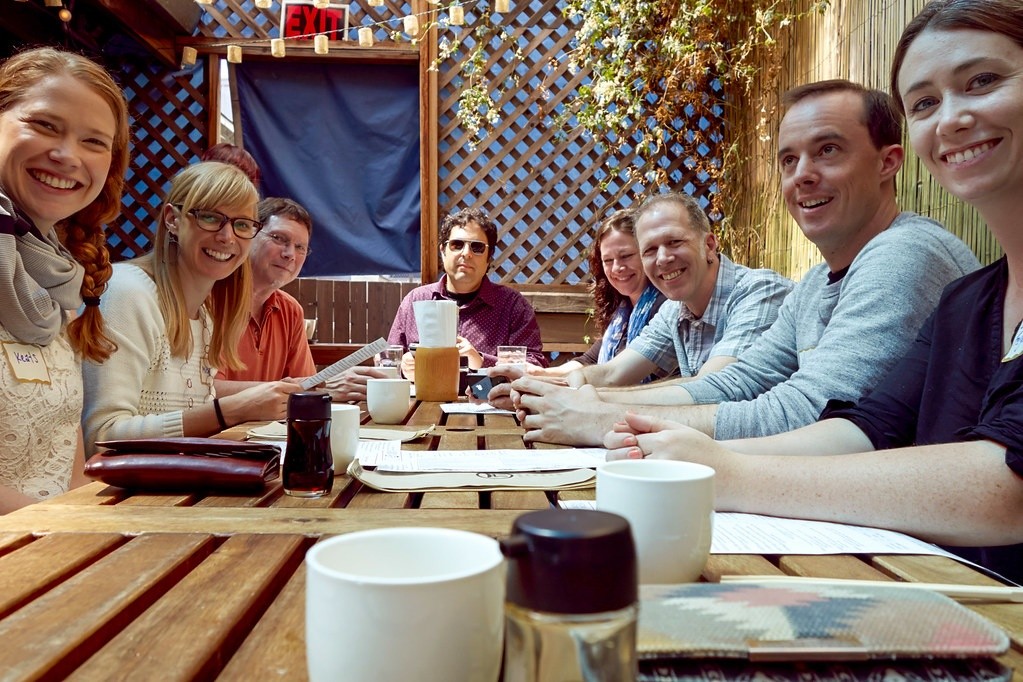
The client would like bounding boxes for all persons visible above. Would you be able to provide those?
[82,161,326,460]
[604,0,1023,584]
[203,142,259,187]
[510,79,983,446]
[465,194,798,409]
[0,48,130,515]
[374,208,548,382]
[213,198,388,402]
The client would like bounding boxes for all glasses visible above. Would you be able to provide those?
[259,228,312,255]
[441,238,492,255]
[173,203,264,239]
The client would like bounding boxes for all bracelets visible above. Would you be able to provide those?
[212,399,229,429]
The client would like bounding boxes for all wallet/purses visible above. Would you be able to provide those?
[87,434,283,488]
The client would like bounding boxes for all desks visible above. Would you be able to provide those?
[306,343,393,365]
[0,384,1023,682]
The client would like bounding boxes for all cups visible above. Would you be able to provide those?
[596,458,715,584]
[306,527,505,681]
[302,318,317,343]
[325,404,360,475]
[414,346,461,402]
[495,345,527,369]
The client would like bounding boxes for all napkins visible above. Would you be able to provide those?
[413,300,460,348]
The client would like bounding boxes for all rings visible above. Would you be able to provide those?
[455,343,462,348]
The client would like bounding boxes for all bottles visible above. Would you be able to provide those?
[366,344,410,424]
[282,392,335,496]
[497,509,640,681]
